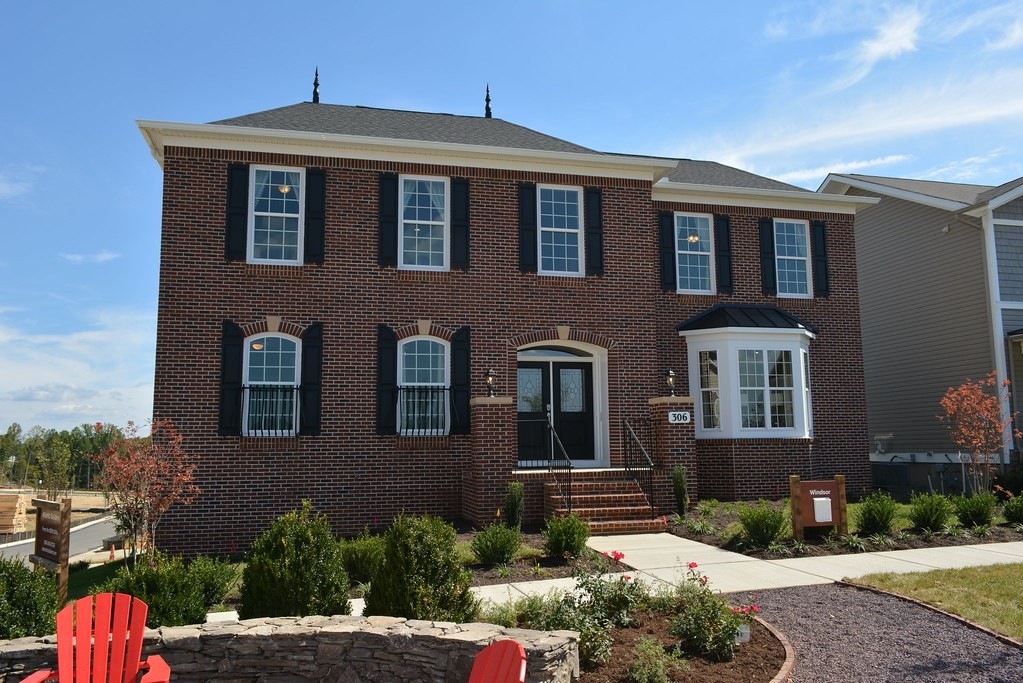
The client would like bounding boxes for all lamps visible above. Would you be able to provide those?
[688,232,698,243]
[252,343,264,350]
[666,369,677,398]
[278,184,291,193]
[484,369,496,397]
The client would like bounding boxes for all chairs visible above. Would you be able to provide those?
[18,590,171,683]
[468,640,528,683]
